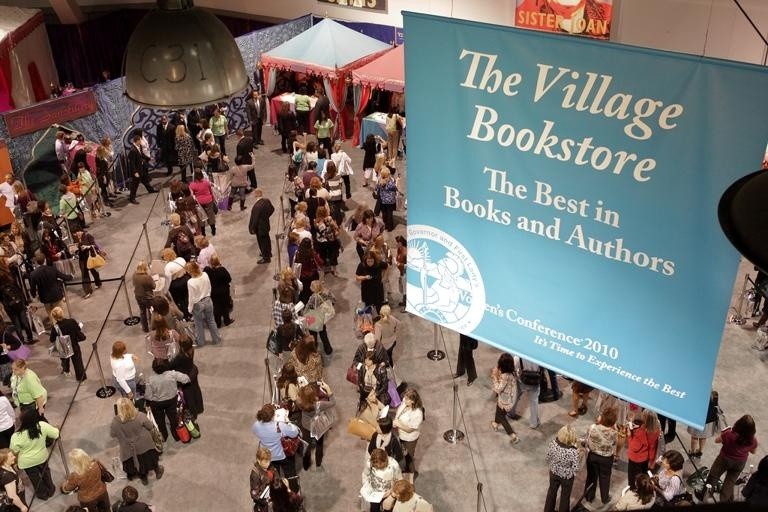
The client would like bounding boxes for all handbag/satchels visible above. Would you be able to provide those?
[101,469,114,482]
[77,211,84,222]
[49,334,74,359]
[666,492,695,506]
[338,159,347,176]
[311,257,322,272]
[374,153,386,173]
[396,120,403,130]
[281,361,339,461]
[86,244,111,269]
[6,335,31,361]
[715,405,727,433]
[53,259,77,278]
[176,419,200,443]
[519,370,541,386]
[165,320,198,364]
[32,315,47,336]
[217,158,229,172]
[287,298,336,352]
[290,262,303,279]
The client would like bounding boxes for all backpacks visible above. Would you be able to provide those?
[175,229,194,257]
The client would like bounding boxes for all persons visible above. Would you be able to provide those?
[512,356,768,512]
[100,69,114,82]
[0,173,100,512]
[248,90,405,512]
[394,389,425,475]
[489,353,519,443]
[60,100,253,512]
[63,81,81,94]
[383,479,434,511]
[751,265,768,328]
[452,334,480,384]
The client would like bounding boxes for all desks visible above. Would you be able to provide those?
[270,92,319,132]
[361,111,405,153]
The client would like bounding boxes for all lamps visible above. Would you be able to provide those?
[124,0,248,105]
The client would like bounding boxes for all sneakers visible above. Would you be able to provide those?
[156,465,164,479]
